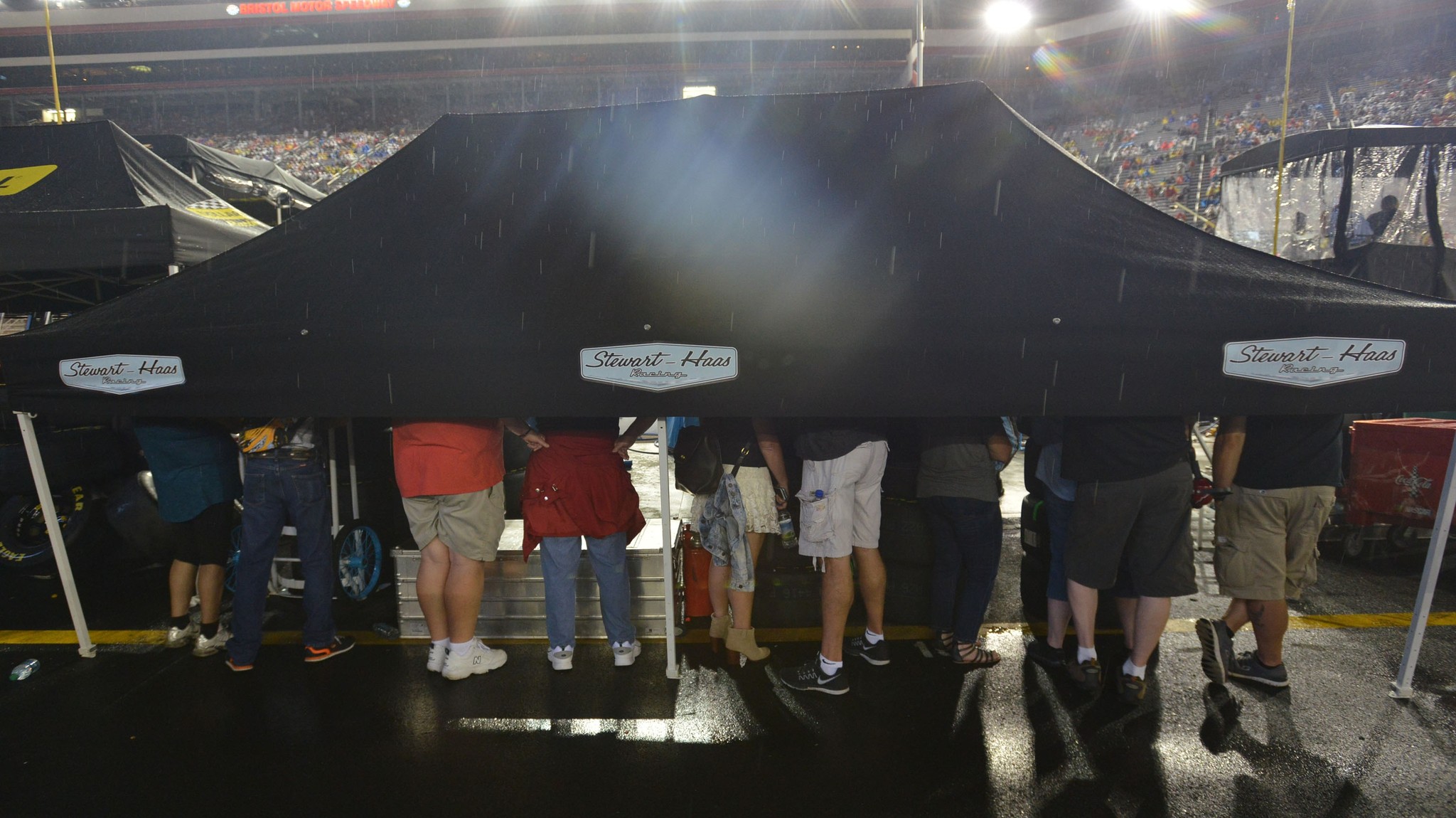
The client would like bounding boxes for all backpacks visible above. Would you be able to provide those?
[674,417,754,495]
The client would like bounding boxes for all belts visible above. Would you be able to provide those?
[247,448,316,460]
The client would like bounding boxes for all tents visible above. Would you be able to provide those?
[0,120,276,314]
[0,81,1456,697]
[133,134,327,224]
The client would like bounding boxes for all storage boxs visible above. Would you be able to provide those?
[389,518,686,640]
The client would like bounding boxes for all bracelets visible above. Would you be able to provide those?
[518,427,531,437]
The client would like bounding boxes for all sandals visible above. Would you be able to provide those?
[934,630,956,651]
[952,637,1000,663]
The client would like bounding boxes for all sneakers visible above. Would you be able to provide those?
[165,614,202,648]
[547,645,574,669]
[193,621,236,657]
[779,651,851,694]
[305,635,356,661]
[611,638,641,666]
[1226,648,1290,686]
[426,639,449,672]
[1196,619,1234,683]
[842,634,890,665]
[225,653,254,671]
[442,637,508,681]
[1065,656,1104,693]
[1114,663,1145,706]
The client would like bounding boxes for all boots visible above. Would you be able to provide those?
[725,623,771,664]
[708,612,736,653]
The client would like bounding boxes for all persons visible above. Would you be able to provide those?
[224,416,356,671]
[391,418,506,680]
[1197,413,1345,687]
[1025,415,1196,705]
[702,417,790,664]
[778,415,891,695]
[502,417,656,670]
[114,70,1455,259]
[132,415,238,657]
[915,415,1012,664]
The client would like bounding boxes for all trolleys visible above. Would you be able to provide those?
[241,417,382,602]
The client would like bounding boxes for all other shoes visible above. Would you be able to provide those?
[1028,637,1068,668]
[1116,644,1136,661]
